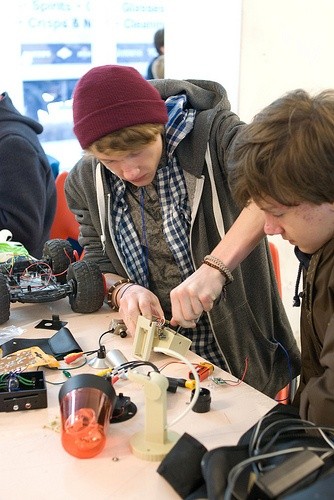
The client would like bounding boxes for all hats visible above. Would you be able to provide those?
[72,65,169,150]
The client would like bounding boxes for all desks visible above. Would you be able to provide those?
[0,295,280,500]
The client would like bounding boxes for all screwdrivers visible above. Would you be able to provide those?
[175,326,181,334]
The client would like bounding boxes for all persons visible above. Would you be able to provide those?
[146,29,164,78]
[228,87,334,438]
[64,64,302,400]
[0,92,57,261]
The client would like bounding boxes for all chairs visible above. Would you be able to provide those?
[49,171,297,403]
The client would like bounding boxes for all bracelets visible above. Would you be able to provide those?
[200,255,234,284]
[106,278,137,312]
